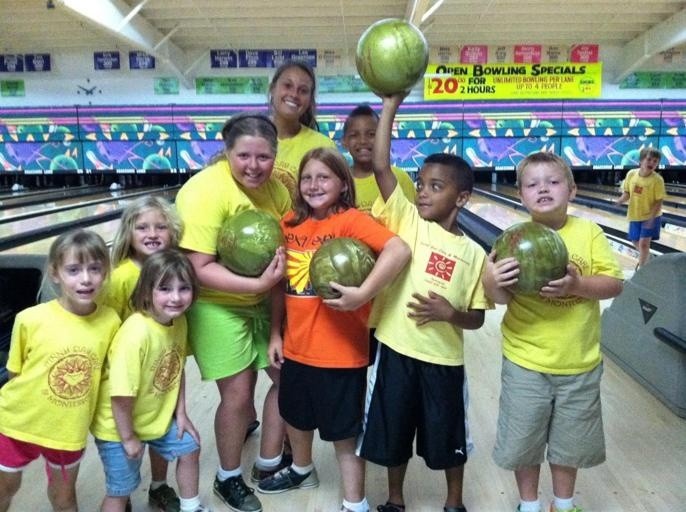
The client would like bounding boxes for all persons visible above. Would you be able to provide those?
[352,92,495,512]
[0,229,123,512]
[90,248,211,512]
[614,148,668,269]
[245,60,338,446]
[95,195,180,512]
[340,104,416,216]
[172,111,292,512]
[481,151,625,512]
[252,147,412,512]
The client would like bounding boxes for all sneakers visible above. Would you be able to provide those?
[148,483,180,511]
[339,497,405,512]
[550,500,582,512]
[253,455,319,494]
[213,473,262,512]
